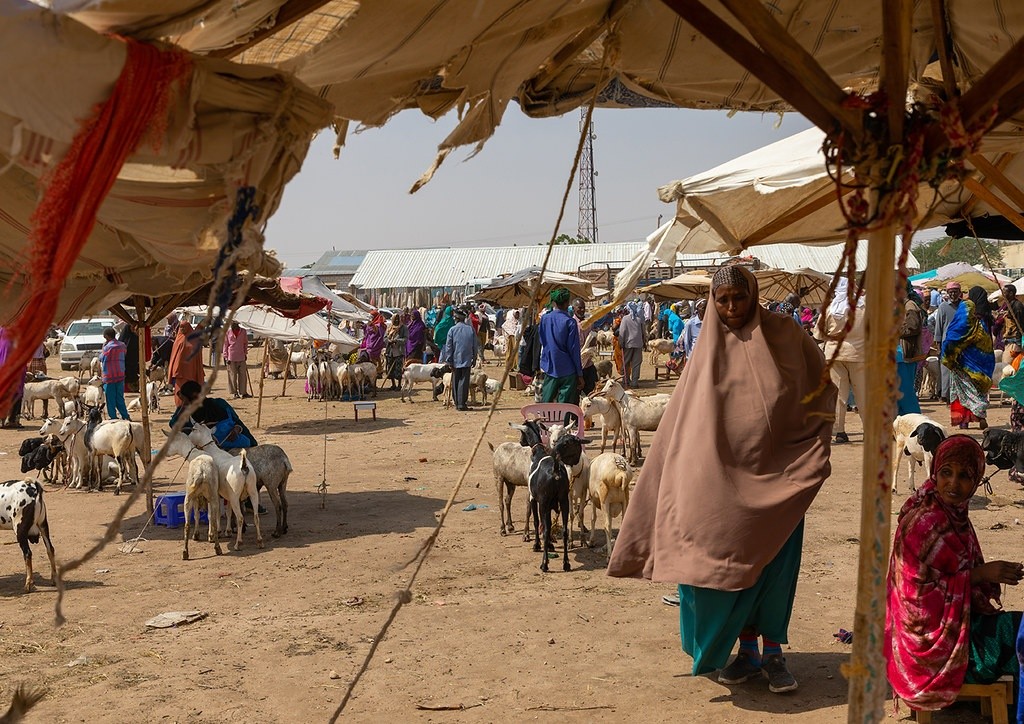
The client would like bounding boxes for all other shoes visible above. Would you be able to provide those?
[835,432,848,443]
[245,501,267,515]
[234,394,252,398]
[958,422,967,429]
[979,419,988,429]
[388,385,401,391]
[456,405,474,411]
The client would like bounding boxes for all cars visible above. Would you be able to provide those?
[60,318,121,372]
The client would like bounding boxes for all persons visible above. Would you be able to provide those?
[934,282,995,430]
[572,298,599,395]
[683,297,708,360]
[537,286,585,427]
[632,296,696,352]
[618,301,648,390]
[769,293,819,336]
[445,310,479,411]
[170,379,268,514]
[268,338,298,380]
[360,300,526,391]
[0,324,50,429]
[223,321,255,399]
[612,308,631,384]
[99,312,204,421]
[884,433,1024,711]
[813,269,943,445]
[606,267,839,695]
[994,284,1024,357]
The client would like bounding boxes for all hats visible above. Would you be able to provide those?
[181,380,201,398]
[103,328,117,336]
[946,282,960,289]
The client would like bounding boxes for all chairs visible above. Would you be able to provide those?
[521,403,585,451]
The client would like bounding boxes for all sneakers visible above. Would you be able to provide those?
[718,654,762,685]
[762,655,797,692]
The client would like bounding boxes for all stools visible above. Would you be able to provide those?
[354,402,376,422]
[599,351,614,361]
[154,493,194,528]
[509,372,525,390]
[655,365,680,379]
[910,676,1014,724]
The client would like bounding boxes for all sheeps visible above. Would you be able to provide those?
[578,373,672,467]
[596,329,615,357]
[644,320,676,366]
[160,414,294,562]
[982,428,1024,473]
[283,339,380,403]
[400,358,502,410]
[892,413,946,493]
[16,329,160,497]
[486,415,635,574]
[483,341,507,368]
[0,477,59,595]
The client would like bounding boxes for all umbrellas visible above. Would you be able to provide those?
[640,271,713,301]
[181,271,376,429]
[472,265,594,324]
[753,267,832,311]
[105,262,303,526]
